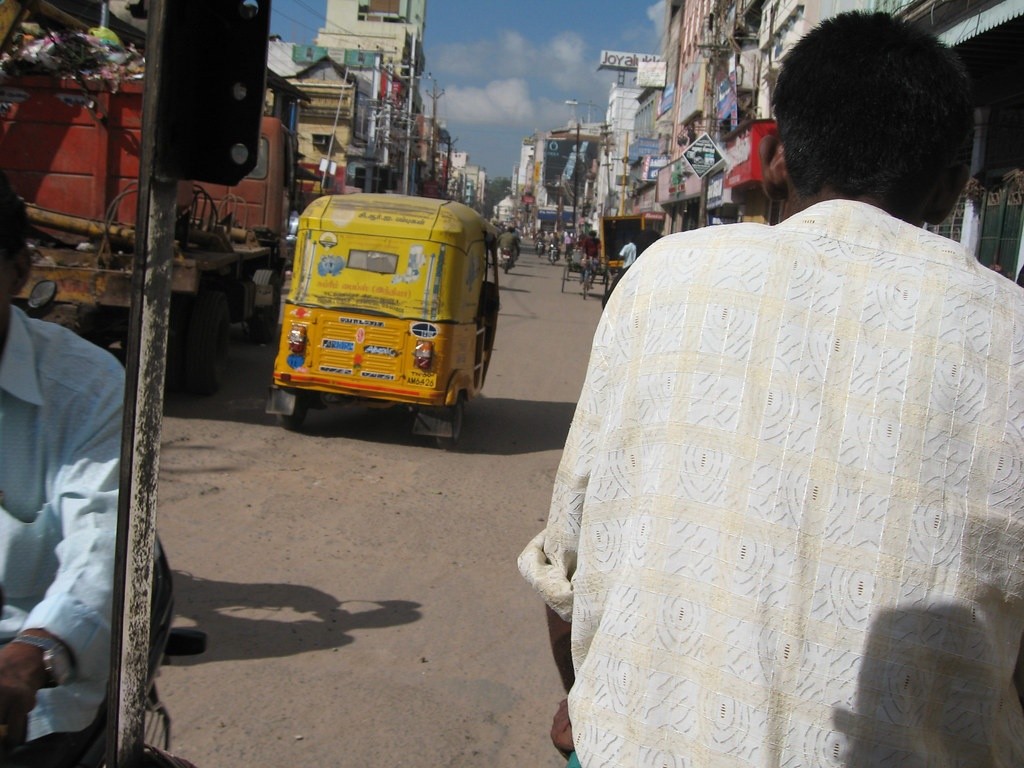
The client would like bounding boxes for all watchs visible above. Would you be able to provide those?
[14,635,72,688]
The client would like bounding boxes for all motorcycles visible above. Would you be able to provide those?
[264,192,501,450]
[546,243,563,266]
[499,240,517,275]
[534,236,545,258]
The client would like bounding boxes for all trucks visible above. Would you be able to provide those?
[0,0,315,397]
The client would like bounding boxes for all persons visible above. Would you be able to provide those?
[603,229,662,310]
[520,14,1024,768]
[0,183,130,768]
[497,226,520,256]
[534,228,602,271]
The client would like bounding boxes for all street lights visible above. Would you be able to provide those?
[563,100,613,217]
[550,123,580,245]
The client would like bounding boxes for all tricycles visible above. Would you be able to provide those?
[560,253,602,301]
